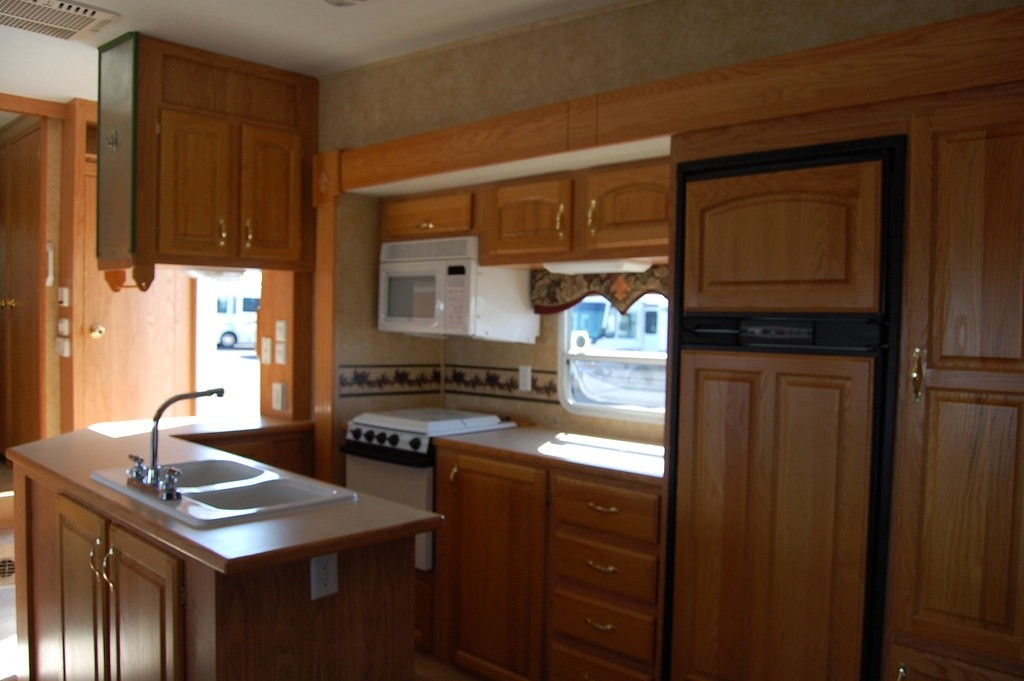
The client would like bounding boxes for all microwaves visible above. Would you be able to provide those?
[378,236,544,348]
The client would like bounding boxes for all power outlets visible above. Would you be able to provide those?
[310,553,338,602]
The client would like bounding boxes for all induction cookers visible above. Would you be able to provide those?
[346,407,516,466]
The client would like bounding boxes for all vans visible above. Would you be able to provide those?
[218,296,260,349]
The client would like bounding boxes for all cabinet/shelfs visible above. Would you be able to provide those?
[545,471,669,680]
[53,491,185,681]
[680,162,883,313]
[671,347,874,680]
[154,101,303,261]
[879,94,1024,681]
[486,164,670,255]
[435,445,547,681]
